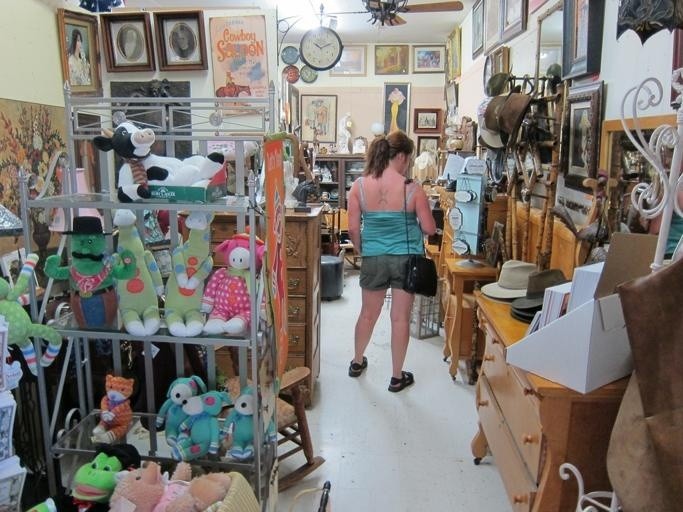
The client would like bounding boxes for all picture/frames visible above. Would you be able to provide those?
[285,30,462,161]
[595,113,682,228]
[560,79,609,192]
[98,6,157,75]
[151,8,207,72]
[469,0,615,91]
[55,7,101,98]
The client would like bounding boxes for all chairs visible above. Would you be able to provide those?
[130,293,328,502]
[324,206,356,267]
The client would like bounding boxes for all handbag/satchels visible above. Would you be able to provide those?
[403,257,439,298]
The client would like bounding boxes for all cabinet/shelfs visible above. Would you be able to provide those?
[15,80,283,512]
[177,208,323,407]
[310,153,368,268]
[468,288,632,512]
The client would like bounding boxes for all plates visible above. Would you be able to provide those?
[346,169,364,173]
[328,188,338,199]
[282,66,299,83]
[300,64,317,83]
[280,46,298,64]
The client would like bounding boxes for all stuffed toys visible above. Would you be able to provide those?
[199,226,264,338]
[155,372,206,450]
[161,209,216,339]
[88,372,136,447]
[220,383,278,461]
[0,252,62,380]
[41,214,138,331]
[88,115,227,206]
[107,455,264,511]
[28,440,145,511]
[178,388,234,461]
[110,207,168,340]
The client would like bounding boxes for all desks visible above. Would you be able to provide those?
[440,258,500,383]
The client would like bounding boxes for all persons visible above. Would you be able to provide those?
[121,28,143,63]
[385,86,405,133]
[66,25,93,88]
[171,27,195,61]
[346,129,440,393]
[77,130,97,193]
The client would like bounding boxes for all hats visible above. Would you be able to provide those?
[481,260,572,324]
[477,62,534,152]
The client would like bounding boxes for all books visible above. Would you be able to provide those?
[0,314,27,511]
[523,257,607,335]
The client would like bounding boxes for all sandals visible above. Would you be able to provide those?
[348,356,368,378]
[389,370,416,393]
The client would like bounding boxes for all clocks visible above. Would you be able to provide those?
[297,4,344,72]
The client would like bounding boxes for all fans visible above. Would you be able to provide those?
[305,0,468,32]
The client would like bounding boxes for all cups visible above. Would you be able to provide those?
[321,192,330,201]
